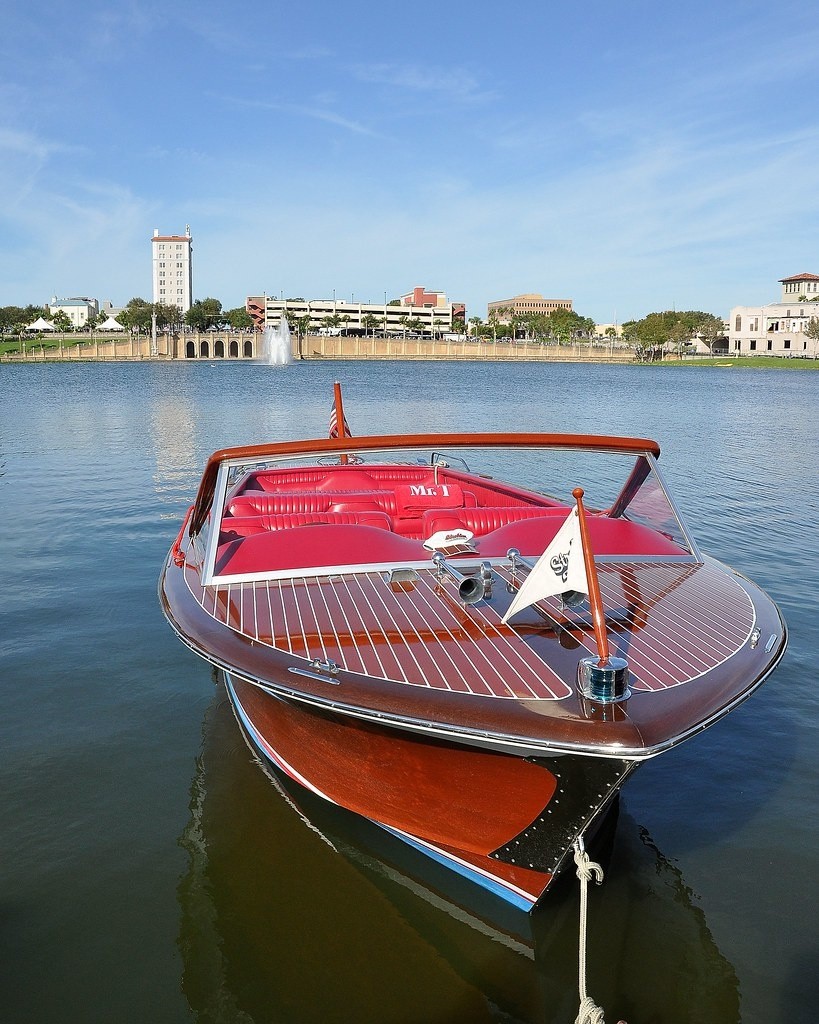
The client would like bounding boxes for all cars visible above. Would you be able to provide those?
[686,350,696,355]
[480,335,492,343]
[469,337,480,343]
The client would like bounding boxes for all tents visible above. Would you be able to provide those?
[95,316,125,332]
[24,317,54,334]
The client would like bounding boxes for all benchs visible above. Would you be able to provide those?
[422,506,594,540]
[219,511,393,545]
[227,489,478,540]
[247,466,447,491]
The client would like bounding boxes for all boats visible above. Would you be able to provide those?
[158,380,787,909]
[163,691,743,1024]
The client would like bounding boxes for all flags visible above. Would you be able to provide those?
[329,398,353,438]
